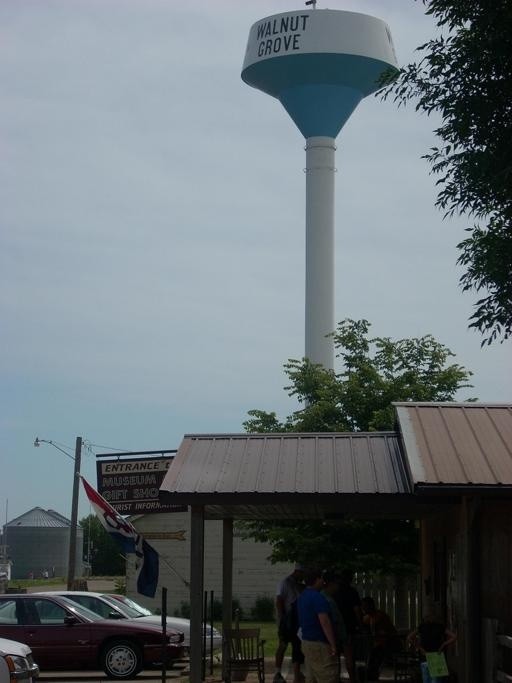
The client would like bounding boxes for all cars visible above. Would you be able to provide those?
[0,592,223,683]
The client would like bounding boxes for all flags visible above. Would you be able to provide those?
[81,475,159,598]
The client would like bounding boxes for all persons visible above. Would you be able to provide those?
[273,567,458,683]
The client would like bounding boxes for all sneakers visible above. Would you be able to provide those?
[292,674,305,681]
[273,672,286,683]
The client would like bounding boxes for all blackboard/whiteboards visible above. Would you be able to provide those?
[99,456,188,514]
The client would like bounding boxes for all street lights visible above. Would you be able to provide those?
[33,435,83,591]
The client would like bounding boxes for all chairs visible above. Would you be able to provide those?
[222,627,266,683]
[355,623,423,683]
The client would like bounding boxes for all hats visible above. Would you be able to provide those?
[295,562,304,571]
